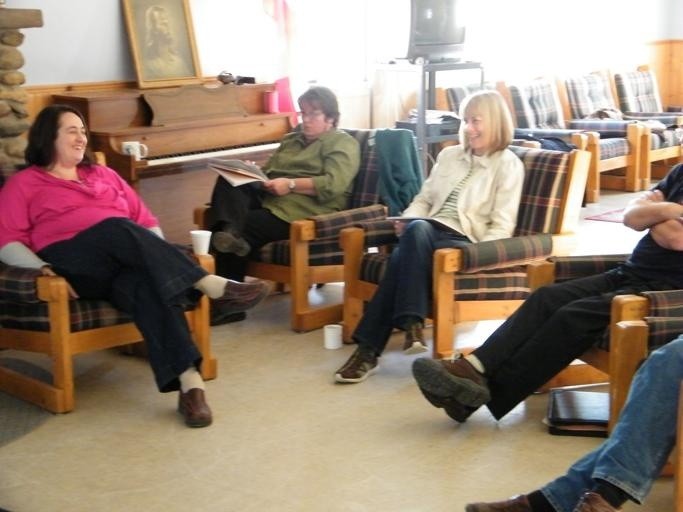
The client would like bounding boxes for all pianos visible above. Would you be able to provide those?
[52,82,297,244]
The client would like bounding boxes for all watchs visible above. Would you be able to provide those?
[287,177,297,193]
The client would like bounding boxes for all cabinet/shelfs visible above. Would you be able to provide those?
[368,62,485,179]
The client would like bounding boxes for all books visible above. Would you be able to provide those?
[207,154,270,187]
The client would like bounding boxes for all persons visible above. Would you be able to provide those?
[0,105,270,428]
[332,90,525,382]
[414,163,683,422]
[458,334,683,512]
[209,85,362,325]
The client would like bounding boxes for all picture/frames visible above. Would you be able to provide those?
[120,1,204,91]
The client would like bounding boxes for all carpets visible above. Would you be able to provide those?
[584,208,626,223]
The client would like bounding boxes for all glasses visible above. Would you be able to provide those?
[297,111,323,117]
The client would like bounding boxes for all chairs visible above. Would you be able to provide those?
[562,68,683,191]
[0,151,216,414]
[608,317,682,511]
[527,255,682,395]
[435,82,587,154]
[609,64,683,118]
[194,128,420,332]
[339,141,595,359]
[496,76,641,202]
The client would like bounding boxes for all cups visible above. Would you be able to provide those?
[324,324,344,349]
[264,90,279,114]
[190,230,211,254]
[122,141,147,163]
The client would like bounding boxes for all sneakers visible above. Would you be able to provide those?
[212,232,252,256]
[572,492,624,512]
[403,322,428,353]
[466,494,532,512]
[420,386,478,423]
[335,346,379,383]
[412,353,490,407]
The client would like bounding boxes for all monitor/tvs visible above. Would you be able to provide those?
[410,0,469,65]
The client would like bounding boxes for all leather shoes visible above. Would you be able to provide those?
[209,281,268,321]
[179,386,211,427]
